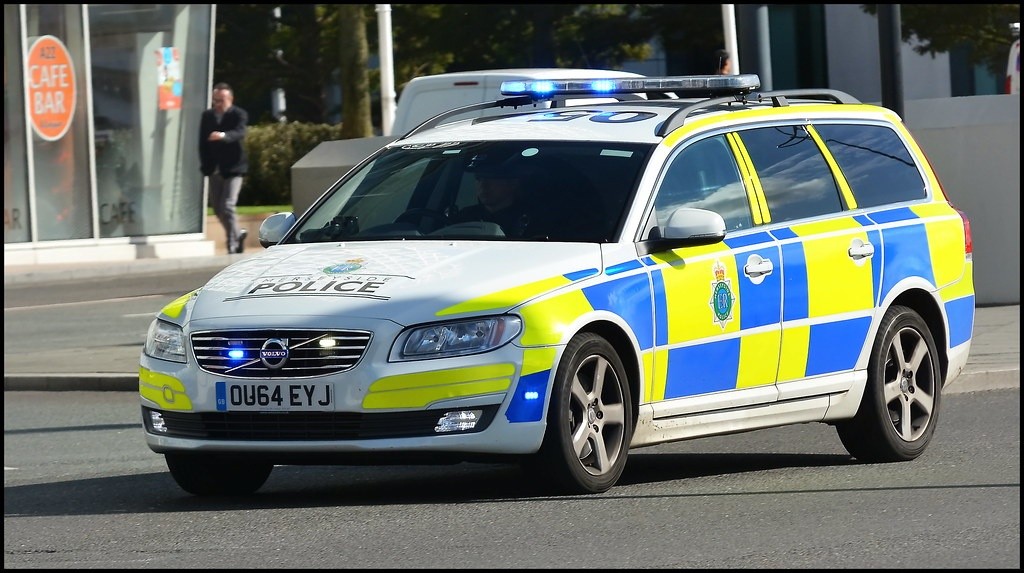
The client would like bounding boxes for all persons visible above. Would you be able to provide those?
[198,83,247,253]
[445,155,548,236]
[711,49,731,75]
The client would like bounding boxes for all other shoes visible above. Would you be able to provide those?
[228,230,247,253]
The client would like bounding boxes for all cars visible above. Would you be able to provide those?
[133,73,978,497]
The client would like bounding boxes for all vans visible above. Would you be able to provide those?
[390,67,680,139]
